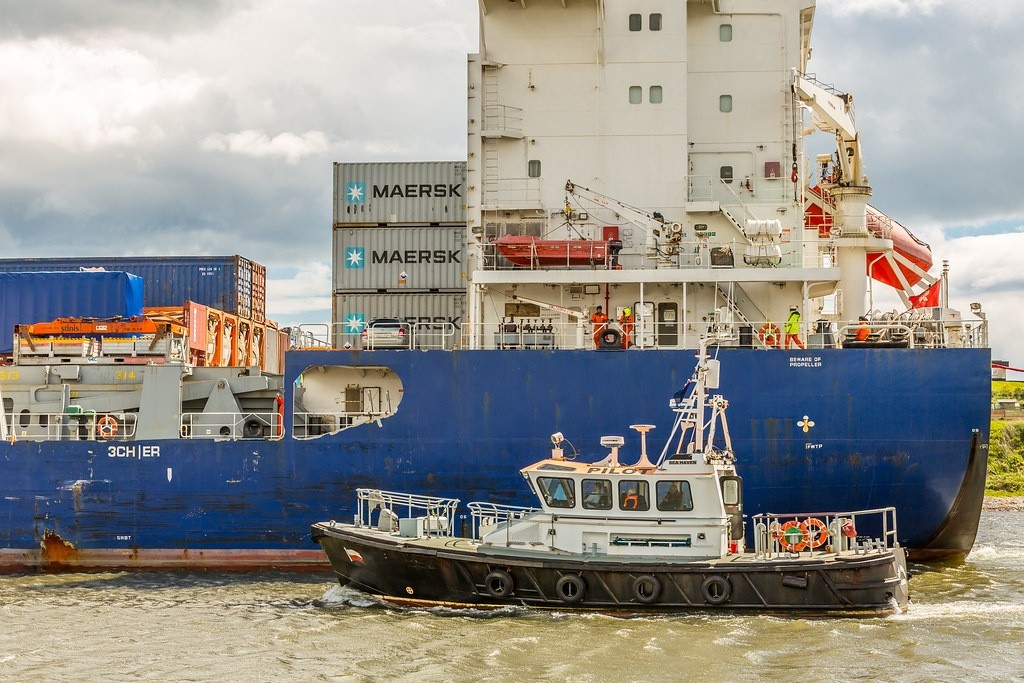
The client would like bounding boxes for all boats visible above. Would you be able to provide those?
[494,233,614,267]
[801,179,934,294]
[310,328,912,626]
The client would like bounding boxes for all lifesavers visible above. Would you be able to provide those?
[777,520,809,553]
[98,417,119,438]
[630,574,662,604]
[599,329,621,349]
[800,518,827,548]
[759,325,781,347]
[554,573,585,604]
[484,570,513,600]
[701,574,732,605]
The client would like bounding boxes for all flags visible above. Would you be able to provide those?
[672,380,692,405]
[910,279,941,310]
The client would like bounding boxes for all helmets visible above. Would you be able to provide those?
[623,307,632,317]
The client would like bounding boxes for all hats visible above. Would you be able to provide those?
[790,306,797,309]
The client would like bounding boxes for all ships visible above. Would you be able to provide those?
[0,0,993,573]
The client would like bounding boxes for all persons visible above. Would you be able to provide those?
[598,485,608,507]
[783,305,804,349]
[659,483,681,509]
[589,305,606,349]
[620,306,634,349]
[584,481,602,508]
[623,488,640,508]
[854,316,871,340]
